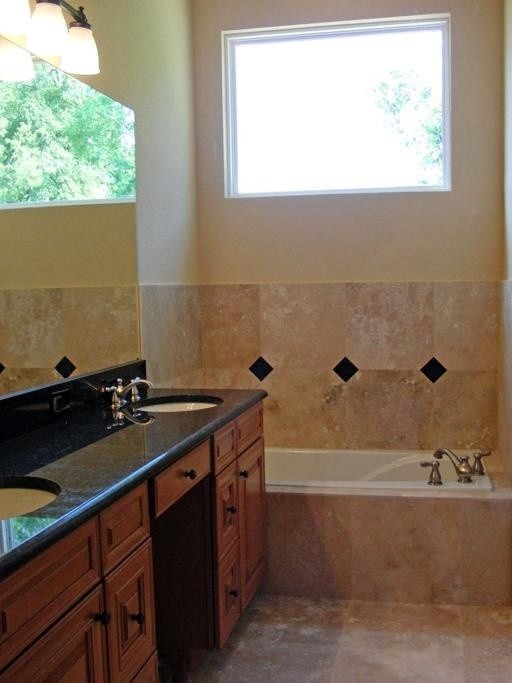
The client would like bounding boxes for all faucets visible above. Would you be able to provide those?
[104,376,154,403]
[106,404,155,430]
[433,448,473,482]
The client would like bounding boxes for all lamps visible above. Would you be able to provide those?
[30,0,99,75]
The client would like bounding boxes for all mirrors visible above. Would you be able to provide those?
[0,35,142,396]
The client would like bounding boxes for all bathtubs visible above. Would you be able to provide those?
[259,446,492,496]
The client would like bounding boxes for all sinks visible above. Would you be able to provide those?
[0,473,62,521]
[132,393,227,420]
